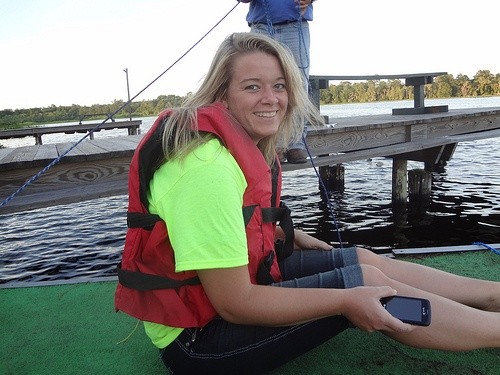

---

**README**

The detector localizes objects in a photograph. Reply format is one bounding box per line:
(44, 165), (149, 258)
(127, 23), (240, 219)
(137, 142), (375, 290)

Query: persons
(110, 34), (500, 375)
(239, 0), (315, 100)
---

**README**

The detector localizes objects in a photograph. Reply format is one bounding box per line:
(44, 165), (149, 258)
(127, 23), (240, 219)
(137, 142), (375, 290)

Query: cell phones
(380, 295), (432, 327)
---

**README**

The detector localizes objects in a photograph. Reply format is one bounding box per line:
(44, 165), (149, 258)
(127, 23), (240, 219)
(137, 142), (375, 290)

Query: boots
(275, 148), (286, 163)
(288, 148), (308, 162)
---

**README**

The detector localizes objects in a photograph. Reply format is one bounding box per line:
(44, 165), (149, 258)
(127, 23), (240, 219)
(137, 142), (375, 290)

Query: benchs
(78, 113), (115, 124)
(307, 72), (448, 125)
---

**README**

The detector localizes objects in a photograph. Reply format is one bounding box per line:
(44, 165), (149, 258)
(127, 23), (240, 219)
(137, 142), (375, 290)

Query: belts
(254, 19), (308, 26)
